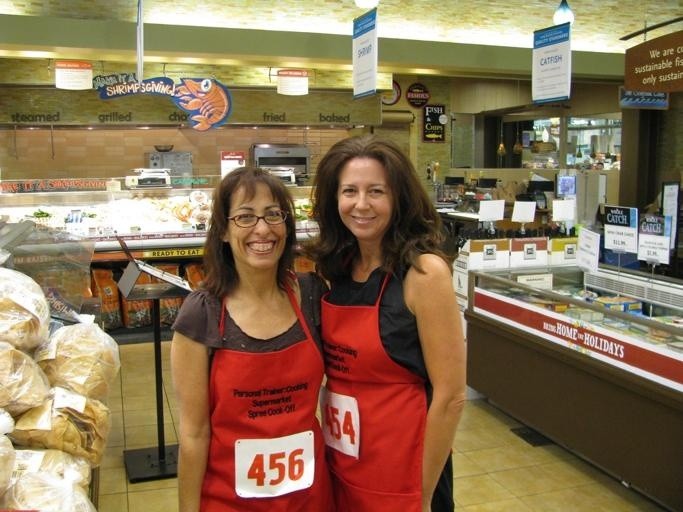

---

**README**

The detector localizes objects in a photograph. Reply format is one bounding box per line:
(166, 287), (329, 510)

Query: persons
(307, 132), (468, 511)
(167, 165), (334, 511)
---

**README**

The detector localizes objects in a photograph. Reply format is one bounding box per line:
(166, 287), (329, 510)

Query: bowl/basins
(153, 144), (173, 152)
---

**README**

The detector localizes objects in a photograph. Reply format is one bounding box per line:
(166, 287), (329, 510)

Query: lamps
(496, 114), (612, 159)
(553, 0), (575, 27)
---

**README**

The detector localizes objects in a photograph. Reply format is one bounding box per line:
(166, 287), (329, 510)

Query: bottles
(432, 160), (439, 183)
(455, 227), (577, 253)
(425, 164), (431, 181)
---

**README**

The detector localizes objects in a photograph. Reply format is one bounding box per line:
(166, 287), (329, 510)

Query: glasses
(227, 209), (290, 228)
